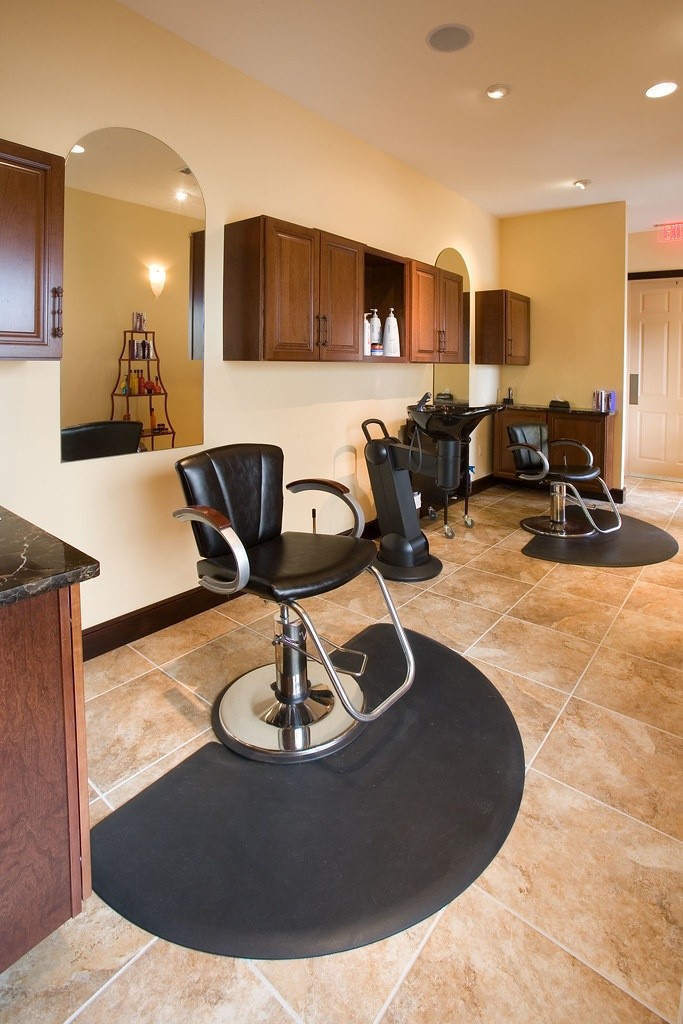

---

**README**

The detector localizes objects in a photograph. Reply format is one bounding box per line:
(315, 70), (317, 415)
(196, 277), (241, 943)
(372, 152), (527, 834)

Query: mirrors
(434, 248), (471, 408)
(61, 128), (206, 462)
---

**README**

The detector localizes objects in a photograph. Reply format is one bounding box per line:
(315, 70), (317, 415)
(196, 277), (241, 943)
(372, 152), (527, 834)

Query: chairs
(506, 420), (622, 538)
(173, 443), (415, 766)
(61, 421), (143, 463)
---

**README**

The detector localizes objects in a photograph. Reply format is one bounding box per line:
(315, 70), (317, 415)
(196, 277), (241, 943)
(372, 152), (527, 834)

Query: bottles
(150, 408), (156, 432)
(129, 370), (154, 394)
(120, 375), (131, 395)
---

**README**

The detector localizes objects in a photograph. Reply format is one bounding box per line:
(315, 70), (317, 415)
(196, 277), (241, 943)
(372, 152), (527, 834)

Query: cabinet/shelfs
(475, 289), (530, 366)
(222, 215), (464, 363)
(0, 139), (65, 359)
(110, 328), (177, 452)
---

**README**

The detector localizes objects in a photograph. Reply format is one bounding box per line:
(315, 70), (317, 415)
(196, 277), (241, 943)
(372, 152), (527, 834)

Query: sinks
(407, 404), (507, 441)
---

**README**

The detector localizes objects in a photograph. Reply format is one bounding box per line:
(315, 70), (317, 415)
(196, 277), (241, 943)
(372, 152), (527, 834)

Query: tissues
(436, 388), (453, 401)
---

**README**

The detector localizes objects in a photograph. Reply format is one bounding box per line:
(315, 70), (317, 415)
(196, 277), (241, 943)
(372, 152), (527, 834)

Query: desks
(0, 510), (101, 975)
(494, 405), (616, 494)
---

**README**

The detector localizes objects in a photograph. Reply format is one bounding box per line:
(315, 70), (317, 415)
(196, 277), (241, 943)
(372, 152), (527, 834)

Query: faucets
(415, 392), (432, 412)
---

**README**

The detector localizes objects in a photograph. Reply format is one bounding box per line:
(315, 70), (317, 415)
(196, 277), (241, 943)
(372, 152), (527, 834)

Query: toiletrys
(363, 308), (401, 357)
